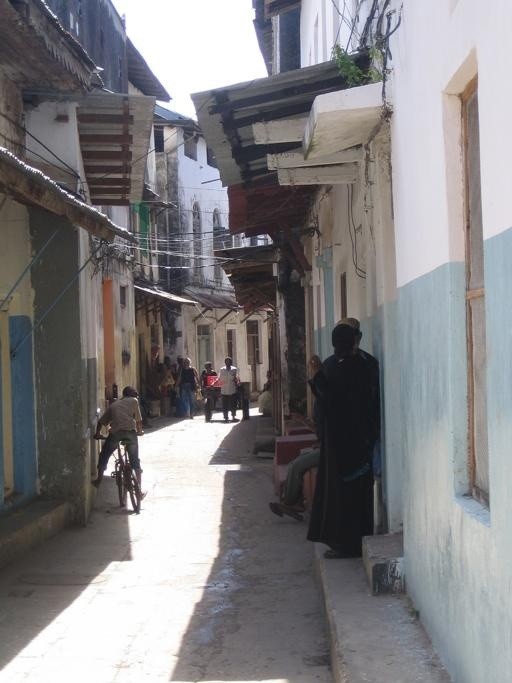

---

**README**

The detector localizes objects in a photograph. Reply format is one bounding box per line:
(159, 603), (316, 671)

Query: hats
(332, 318), (361, 331)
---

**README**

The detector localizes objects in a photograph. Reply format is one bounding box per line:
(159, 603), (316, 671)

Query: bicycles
(93, 431), (144, 514)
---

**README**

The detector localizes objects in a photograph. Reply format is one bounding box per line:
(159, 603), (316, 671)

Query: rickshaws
(202, 382), (250, 421)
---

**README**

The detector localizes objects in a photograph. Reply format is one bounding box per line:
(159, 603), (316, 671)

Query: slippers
(269, 502), (304, 521)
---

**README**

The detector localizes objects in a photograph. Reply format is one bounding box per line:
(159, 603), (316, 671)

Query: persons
(268, 409), (322, 520)
(91, 383), (148, 500)
(305, 322), (380, 560)
(312, 315), (380, 536)
(144, 354), (273, 423)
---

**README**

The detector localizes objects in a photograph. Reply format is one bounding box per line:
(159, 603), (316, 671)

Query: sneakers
(323, 547), (342, 559)
(92, 480), (100, 487)
(141, 490), (147, 499)
